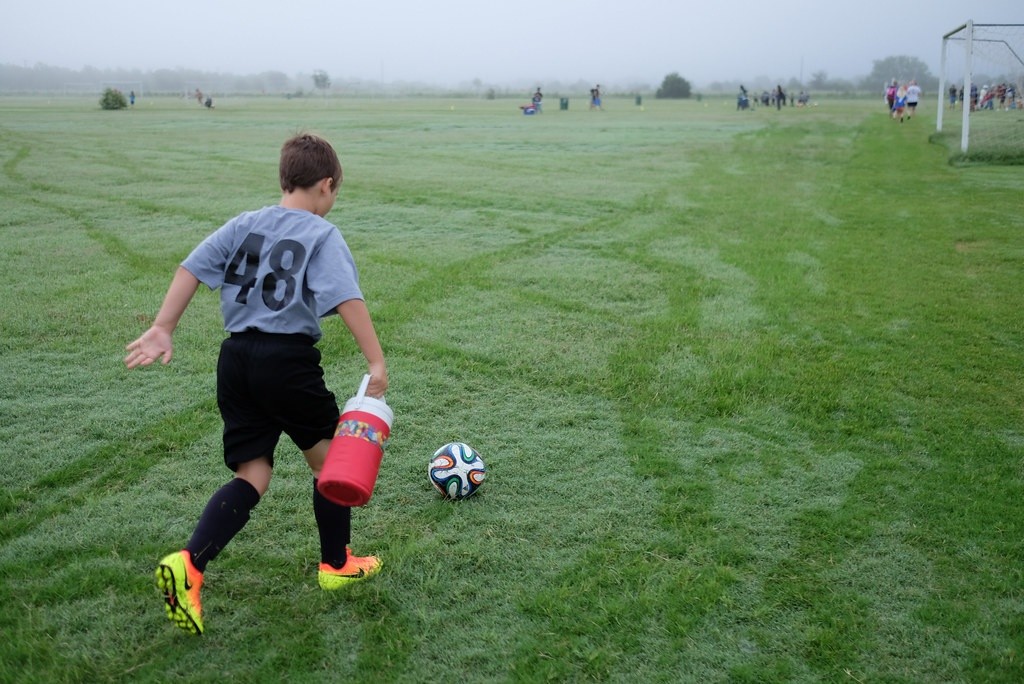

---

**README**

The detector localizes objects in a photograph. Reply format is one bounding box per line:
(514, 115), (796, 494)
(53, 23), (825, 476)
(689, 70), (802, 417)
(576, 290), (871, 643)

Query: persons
(126, 134), (388, 636)
(533, 87), (543, 114)
(195, 87), (204, 104)
(886, 78), (922, 122)
(205, 94), (212, 109)
(129, 91), (135, 111)
(949, 81), (1016, 111)
(590, 85), (605, 110)
(736, 85), (811, 111)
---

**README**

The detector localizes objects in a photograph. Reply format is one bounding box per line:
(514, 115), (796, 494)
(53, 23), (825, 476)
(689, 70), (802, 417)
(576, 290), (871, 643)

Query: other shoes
(1006, 108), (1008, 112)
(901, 118), (903, 123)
(908, 116), (910, 120)
(997, 109), (1000, 112)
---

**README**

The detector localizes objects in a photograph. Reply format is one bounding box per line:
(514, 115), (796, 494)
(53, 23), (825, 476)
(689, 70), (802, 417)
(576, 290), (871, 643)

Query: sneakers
(318, 546), (384, 590)
(154, 549), (206, 636)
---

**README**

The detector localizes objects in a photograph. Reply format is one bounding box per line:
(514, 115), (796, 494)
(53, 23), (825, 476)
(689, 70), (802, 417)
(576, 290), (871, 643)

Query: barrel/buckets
(318, 373), (394, 506)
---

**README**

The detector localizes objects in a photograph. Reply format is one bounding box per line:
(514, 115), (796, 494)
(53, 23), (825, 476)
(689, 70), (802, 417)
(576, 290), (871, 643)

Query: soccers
(426, 441), (487, 501)
